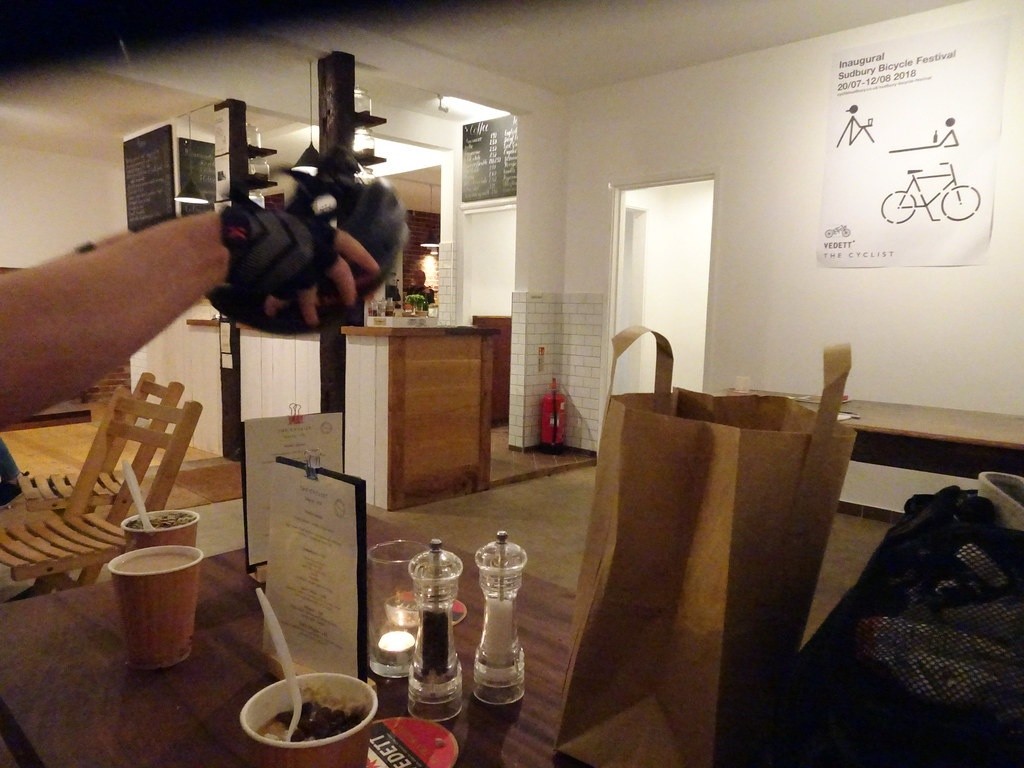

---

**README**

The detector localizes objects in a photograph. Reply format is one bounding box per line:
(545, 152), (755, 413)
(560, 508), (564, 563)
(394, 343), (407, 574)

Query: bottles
(354, 85), (371, 115)
(250, 155), (269, 181)
(368, 296), (394, 317)
(356, 165), (375, 185)
(246, 122), (261, 147)
(249, 188), (265, 210)
(473, 531), (527, 706)
(408, 539), (463, 722)
(354, 125), (374, 155)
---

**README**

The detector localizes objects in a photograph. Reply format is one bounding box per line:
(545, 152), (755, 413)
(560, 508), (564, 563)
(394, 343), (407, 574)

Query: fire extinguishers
(540, 376), (568, 454)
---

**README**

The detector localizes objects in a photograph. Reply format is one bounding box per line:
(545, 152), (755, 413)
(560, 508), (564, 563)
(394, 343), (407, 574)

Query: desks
(707, 384), (1023, 486)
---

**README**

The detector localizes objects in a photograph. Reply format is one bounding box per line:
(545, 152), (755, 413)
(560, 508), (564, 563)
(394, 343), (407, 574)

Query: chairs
(0, 383), (206, 589)
(0, 372), (188, 521)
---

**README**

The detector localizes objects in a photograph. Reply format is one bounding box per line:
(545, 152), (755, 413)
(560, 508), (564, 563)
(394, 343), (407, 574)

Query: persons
(406, 270), (435, 305)
(0, 196), (382, 443)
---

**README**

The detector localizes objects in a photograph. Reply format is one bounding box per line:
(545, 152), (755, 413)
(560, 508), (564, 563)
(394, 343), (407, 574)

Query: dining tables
(0, 512), (581, 767)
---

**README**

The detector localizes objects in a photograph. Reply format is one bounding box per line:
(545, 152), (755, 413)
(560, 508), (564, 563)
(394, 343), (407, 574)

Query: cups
(240, 672), (378, 768)
(108, 545), (204, 669)
(367, 539), (429, 678)
(122, 510), (200, 547)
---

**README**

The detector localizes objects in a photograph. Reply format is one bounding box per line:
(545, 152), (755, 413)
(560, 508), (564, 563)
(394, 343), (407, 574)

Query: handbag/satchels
(553, 315), (859, 768)
(795, 469), (1023, 768)
